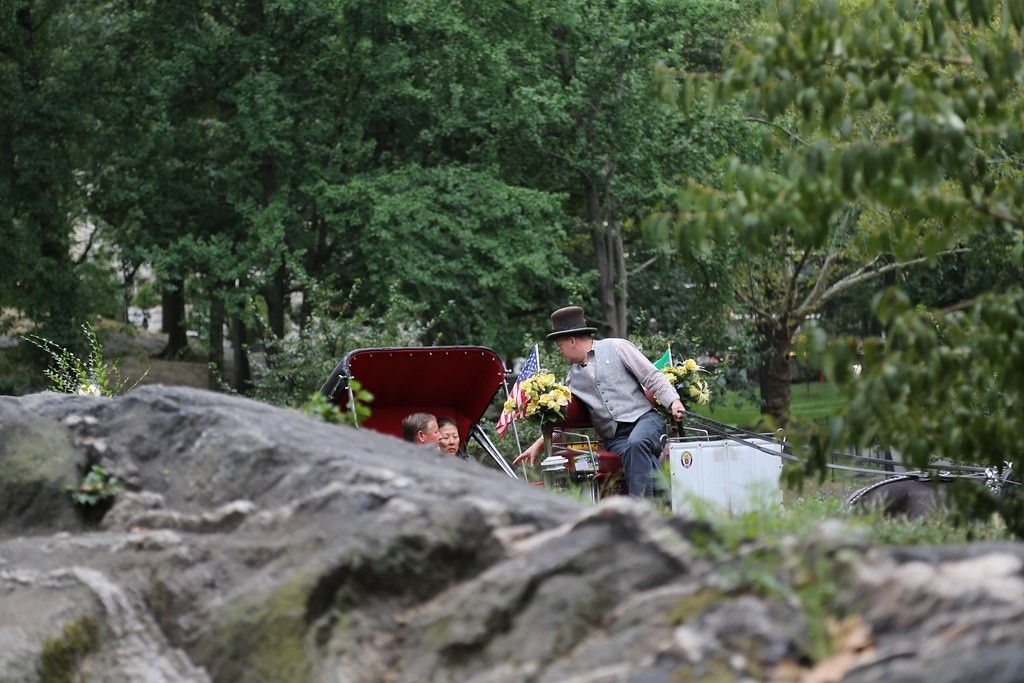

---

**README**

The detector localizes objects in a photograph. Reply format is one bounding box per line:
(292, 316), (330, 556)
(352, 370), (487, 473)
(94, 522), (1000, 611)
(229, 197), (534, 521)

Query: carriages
(312, 345), (1015, 527)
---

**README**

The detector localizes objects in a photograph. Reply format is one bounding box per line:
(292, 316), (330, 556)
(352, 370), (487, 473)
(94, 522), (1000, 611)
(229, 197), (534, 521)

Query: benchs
(364, 407), (472, 454)
(542, 391), (669, 479)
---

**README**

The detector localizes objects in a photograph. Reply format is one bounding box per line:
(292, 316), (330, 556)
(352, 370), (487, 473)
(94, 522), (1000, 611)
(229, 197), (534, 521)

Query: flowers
(506, 376), (573, 430)
(653, 358), (712, 409)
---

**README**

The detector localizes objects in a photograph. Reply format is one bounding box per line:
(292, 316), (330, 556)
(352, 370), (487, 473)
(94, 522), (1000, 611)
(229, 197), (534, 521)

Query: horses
(838, 467), (1024, 540)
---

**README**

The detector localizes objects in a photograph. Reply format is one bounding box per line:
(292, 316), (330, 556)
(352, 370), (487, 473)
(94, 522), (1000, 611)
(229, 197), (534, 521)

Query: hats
(545, 307), (597, 341)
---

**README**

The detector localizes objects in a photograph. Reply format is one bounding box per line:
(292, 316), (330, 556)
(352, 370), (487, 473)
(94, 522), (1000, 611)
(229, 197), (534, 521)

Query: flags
(639, 349), (675, 407)
(496, 343), (539, 437)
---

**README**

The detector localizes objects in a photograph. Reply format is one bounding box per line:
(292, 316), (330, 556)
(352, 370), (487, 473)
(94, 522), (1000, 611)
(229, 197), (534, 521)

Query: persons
(511, 306), (685, 504)
(402, 412), (479, 464)
(696, 349), (723, 365)
